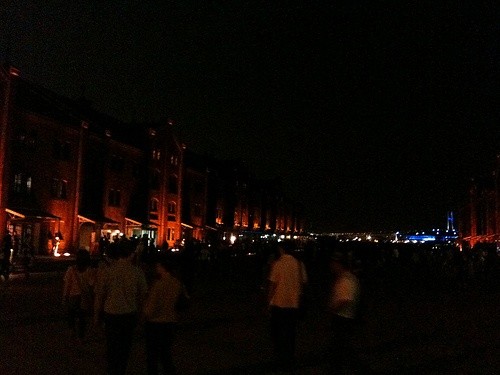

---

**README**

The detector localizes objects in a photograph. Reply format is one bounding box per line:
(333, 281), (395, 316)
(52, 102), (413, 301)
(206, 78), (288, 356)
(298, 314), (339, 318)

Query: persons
(323, 252), (364, 359)
(1, 219), (499, 321)
(267, 238), (309, 359)
(64, 248), (95, 347)
(97, 239), (147, 374)
(141, 249), (182, 374)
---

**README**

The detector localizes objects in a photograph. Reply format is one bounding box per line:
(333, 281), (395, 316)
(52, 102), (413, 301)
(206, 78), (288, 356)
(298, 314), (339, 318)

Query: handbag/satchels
(299, 284), (316, 318)
(176, 294), (191, 316)
(81, 289), (95, 310)
